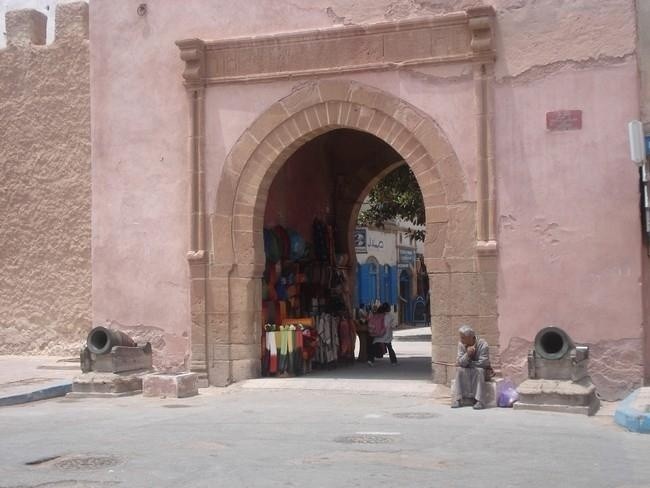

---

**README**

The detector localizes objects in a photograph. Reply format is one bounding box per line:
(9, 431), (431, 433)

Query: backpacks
(367, 311), (389, 337)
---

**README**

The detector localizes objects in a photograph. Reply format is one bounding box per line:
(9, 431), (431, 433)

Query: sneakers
(367, 360), (376, 368)
(391, 361), (401, 367)
(472, 401), (487, 410)
(451, 400), (465, 409)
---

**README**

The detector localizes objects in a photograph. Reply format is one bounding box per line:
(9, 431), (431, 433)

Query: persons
(359, 300), (399, 367)
(448, 325), (496, 409)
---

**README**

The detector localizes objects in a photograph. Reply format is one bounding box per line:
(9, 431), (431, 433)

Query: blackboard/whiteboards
(413, 297), (426, 323)
